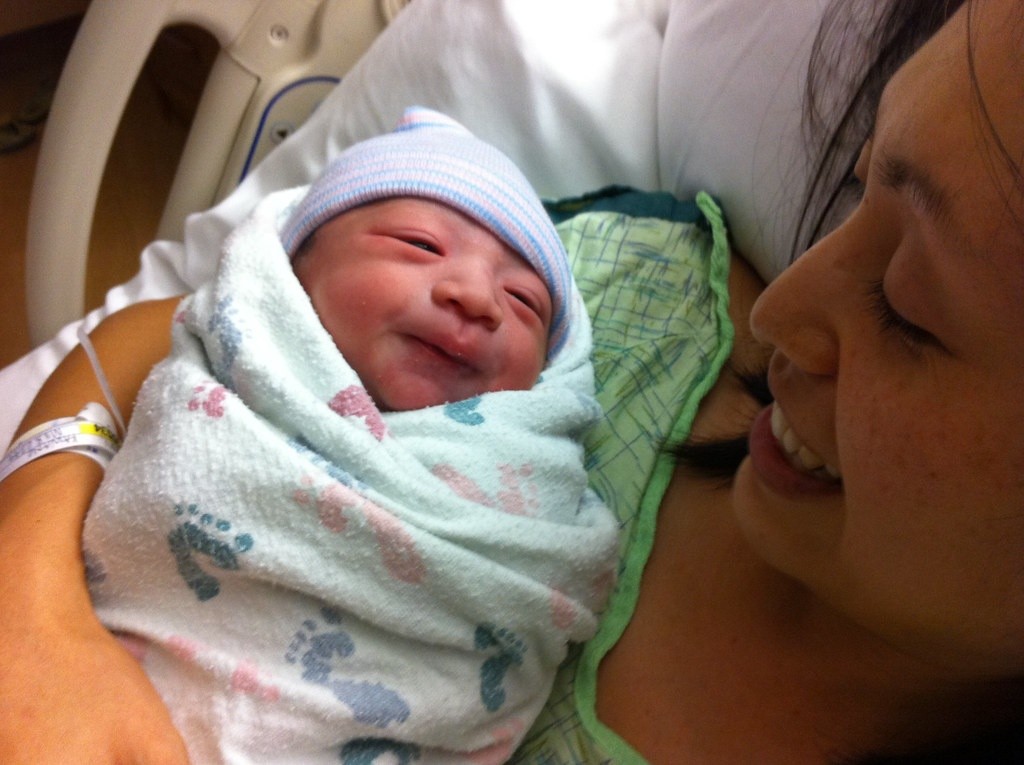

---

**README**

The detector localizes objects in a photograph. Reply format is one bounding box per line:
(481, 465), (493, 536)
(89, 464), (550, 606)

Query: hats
(281, 104), (570, 372)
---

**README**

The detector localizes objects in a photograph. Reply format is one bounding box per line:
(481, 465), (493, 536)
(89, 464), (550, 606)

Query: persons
(0, 0), (1024, 765)
(82, 103), (631, 765)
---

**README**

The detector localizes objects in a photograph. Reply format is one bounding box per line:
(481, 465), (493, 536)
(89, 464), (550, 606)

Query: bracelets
(0, 401), (123, 483)
